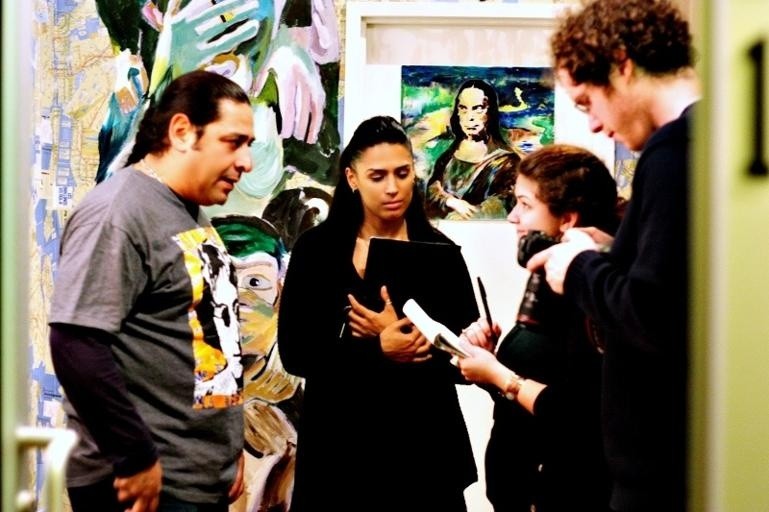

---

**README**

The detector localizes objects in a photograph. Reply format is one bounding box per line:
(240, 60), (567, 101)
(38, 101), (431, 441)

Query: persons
(523, 0), (707, 511)
(447, 146), (628, 511)
(277, 116), (480, 512)
(46, 72), (257, 512)
(423, 78), (522, 221)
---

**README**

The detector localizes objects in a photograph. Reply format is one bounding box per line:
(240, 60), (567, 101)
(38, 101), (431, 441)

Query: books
(402, 299), (469, 359)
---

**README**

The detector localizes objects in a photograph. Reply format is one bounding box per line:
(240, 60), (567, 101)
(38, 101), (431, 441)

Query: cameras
(514, 228), (562, 336)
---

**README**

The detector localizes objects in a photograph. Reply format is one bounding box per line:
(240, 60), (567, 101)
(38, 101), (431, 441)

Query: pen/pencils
(478, 277), (498, 345)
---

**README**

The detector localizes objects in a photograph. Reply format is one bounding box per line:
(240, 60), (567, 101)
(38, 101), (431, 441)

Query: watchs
(504, 376), (525, 400)
